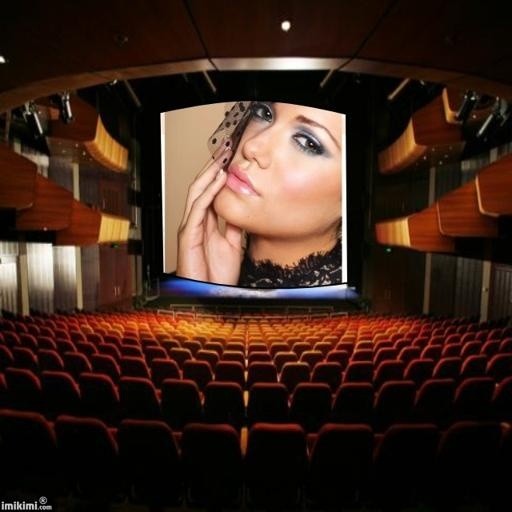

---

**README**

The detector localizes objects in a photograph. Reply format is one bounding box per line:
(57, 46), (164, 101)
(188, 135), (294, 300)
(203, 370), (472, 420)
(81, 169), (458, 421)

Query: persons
(171, 101), (342, 290)
